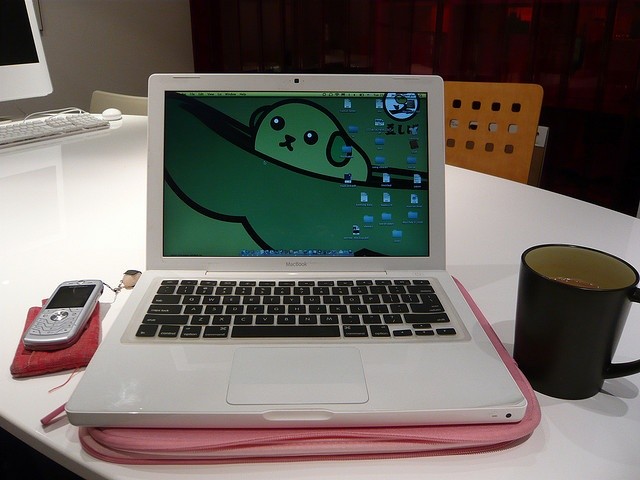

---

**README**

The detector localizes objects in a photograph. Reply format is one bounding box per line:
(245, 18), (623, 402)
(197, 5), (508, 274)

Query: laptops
(64, 73), (528, 429)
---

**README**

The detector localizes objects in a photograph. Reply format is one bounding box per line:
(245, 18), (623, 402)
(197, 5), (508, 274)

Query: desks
(0, 114), (640, 480)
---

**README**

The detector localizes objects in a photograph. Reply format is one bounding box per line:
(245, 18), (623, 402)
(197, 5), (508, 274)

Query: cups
(514, 243), (639, 400)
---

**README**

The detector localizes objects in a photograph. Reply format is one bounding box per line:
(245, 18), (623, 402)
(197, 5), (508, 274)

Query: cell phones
(22, 280), (104, 351)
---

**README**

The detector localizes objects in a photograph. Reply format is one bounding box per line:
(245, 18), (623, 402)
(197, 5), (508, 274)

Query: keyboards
(0, 112), (110, 150)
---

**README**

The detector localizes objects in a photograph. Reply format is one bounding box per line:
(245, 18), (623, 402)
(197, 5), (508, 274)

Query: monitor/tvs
(0, 1), (53, 125)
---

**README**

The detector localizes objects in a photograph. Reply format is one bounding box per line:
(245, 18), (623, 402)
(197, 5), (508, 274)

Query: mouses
(109, 119), (123, 129)
(102, 108), (122, 120)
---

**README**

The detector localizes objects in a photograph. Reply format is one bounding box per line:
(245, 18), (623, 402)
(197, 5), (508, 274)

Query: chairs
(88, 90), (149, 117)
(443, 79), (545, 185)
